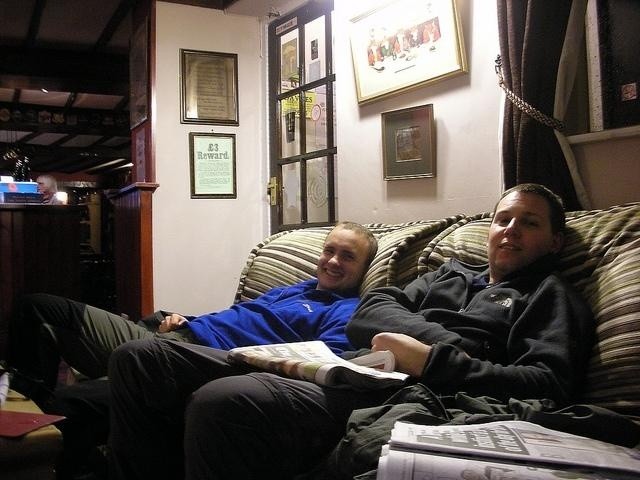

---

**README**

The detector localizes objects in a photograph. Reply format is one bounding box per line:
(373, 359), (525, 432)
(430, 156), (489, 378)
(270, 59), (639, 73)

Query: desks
(0, 385), (66, 479)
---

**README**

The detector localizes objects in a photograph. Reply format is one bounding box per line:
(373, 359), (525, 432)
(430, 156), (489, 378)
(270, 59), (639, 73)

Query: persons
(36, 173), (62, 205)
(14, 219), (379, 454)
(366, 19), (439, 63)
(107, 181), (595, 475)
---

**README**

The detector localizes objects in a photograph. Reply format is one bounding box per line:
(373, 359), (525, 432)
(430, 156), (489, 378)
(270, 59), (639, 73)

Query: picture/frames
(127, 12), (154, 132)
(346, 0), (470, 108)
(176, 47), (240, 128)
(187, 130), (237, 201)
(381, 103), (437, 181)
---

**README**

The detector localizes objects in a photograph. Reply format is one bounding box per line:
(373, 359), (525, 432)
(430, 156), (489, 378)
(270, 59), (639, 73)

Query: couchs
(417, 203), (640, 420)
(65, 213), (467, 419)
